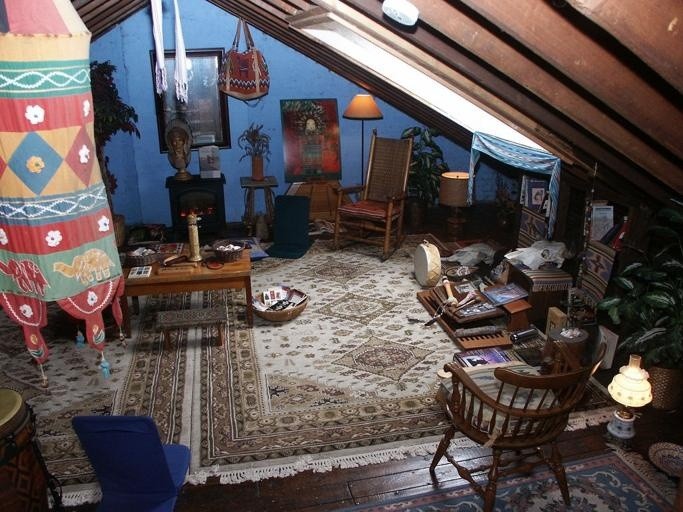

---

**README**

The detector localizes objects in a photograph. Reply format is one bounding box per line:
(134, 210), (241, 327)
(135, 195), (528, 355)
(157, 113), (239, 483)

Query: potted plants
(401, 126), (448, 226)
(238, 122), (272, 181)
(89, 60), (141, 247)
(596, 199), (683, 411)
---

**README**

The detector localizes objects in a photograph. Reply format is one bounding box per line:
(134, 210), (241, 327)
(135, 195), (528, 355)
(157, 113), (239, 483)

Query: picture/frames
(520, 174), (548, 210)
(149, 47), (232, 154)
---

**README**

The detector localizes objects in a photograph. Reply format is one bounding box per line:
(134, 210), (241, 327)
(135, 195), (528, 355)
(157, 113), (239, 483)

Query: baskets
(254, 291), (308, 322)
(213, 240), (245, 262)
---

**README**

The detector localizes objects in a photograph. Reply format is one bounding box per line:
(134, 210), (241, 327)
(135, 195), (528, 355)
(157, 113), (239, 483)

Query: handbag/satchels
(219, 20), (269, 100)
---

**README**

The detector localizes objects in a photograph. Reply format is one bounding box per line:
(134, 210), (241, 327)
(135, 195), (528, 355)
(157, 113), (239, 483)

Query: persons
(168, 131), (191, 167)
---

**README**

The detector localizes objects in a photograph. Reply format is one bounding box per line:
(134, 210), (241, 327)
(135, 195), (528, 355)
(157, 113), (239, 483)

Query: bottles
(510, 327), (538, 343)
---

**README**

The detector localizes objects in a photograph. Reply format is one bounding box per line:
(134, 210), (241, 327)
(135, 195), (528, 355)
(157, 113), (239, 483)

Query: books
(250, 285), (307, 312)
(590, 205), (615, 241)
(454, 347), (507, 368)
(481, 282), (530, 308)
(456, 302), (496, 318)
(519, 175), (550, 215)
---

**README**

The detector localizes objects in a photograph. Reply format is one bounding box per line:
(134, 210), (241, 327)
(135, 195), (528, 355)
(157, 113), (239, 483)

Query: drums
(0, 388), (50, 511)
(414, 242), (441, 287)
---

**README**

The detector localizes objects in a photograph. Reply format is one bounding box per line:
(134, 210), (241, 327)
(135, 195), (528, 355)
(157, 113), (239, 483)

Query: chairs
(72, 415), (190, 512)
(430, 343), (608, 511)
(333, 128), (414, 262)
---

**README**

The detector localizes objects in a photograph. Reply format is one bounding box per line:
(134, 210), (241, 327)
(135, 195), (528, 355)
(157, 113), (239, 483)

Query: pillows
(240, 238), (269, 262)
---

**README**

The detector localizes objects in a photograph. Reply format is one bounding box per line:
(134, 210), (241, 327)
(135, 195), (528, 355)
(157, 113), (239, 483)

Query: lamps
(607, 354), (653, 439)
(341, 95), (383, 186)
(439, 172), (470, 234)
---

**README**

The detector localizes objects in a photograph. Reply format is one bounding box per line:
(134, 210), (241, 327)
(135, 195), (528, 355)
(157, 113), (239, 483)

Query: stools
(156, 308), (226, 352)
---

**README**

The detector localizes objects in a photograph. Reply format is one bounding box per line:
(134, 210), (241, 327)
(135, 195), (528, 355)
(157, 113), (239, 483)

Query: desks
(240, 176), (279, 224)
(122, 242), (255, 338)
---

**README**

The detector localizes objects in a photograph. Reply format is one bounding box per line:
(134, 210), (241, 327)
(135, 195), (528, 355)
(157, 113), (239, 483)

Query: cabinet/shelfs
(165, 173), (226, 239)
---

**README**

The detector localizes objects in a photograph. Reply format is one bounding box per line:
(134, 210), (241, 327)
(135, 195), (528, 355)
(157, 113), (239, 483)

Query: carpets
(191, 233), (644, 484)
(0, 242), (198, 507)
(326, 448), (683, 512)
